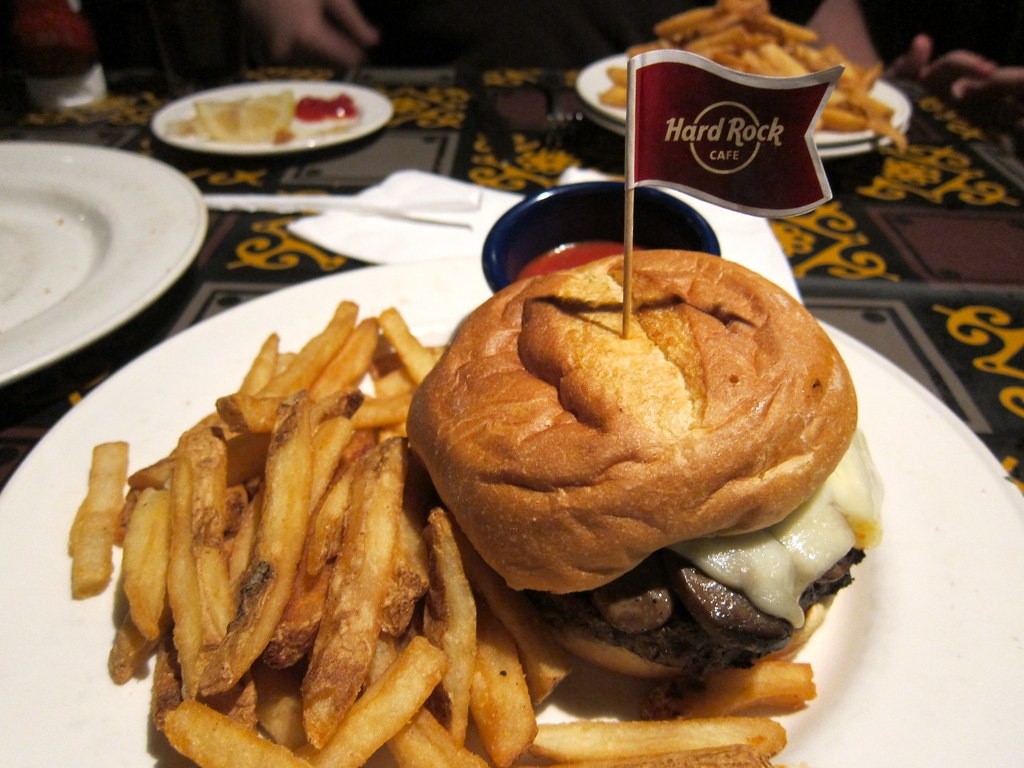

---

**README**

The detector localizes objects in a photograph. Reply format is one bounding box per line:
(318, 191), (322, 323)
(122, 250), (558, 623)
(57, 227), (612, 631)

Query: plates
(0, 254), (1024, 768)
(151, 81), (395, 155)
(0, 140), (209, 383)
(574, 51), (913, 161)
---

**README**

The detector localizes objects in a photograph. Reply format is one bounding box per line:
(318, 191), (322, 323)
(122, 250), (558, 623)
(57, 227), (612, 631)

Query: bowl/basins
(479, 180), (722, 299)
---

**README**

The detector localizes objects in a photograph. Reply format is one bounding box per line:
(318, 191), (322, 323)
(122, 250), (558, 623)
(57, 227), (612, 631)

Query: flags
(633, 49), (846, 220)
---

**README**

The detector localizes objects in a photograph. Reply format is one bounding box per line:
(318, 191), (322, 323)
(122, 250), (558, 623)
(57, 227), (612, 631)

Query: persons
(187, 0), (1024, 136)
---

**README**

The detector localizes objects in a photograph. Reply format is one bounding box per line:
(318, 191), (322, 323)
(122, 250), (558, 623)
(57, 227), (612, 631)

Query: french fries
(599, 1), (910, 149)
(68, 303), (814, 768)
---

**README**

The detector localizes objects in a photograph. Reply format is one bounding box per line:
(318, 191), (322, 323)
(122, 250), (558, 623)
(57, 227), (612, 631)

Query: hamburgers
(406, 248), (881, 680)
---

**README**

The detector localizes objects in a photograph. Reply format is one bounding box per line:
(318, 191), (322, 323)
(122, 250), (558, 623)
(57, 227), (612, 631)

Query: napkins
(286, 179), (524, 266)
(559, 164), (803, 309)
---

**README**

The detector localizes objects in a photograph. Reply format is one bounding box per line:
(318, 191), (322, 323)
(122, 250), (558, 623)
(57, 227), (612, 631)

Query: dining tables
(0, 68), (1023, 767)
